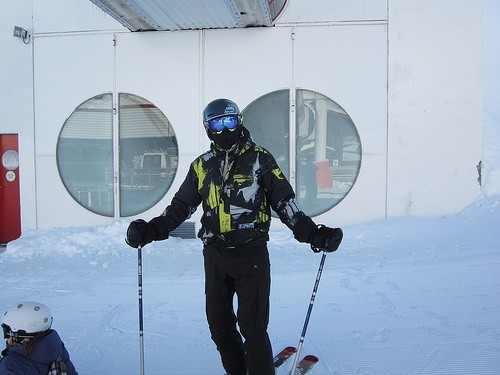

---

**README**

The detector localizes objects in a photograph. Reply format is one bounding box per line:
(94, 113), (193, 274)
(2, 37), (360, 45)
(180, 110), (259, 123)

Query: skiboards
(271, 345), (319, 375)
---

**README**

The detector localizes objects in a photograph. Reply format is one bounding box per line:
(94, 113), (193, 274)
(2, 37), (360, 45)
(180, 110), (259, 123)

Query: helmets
(202, 99), (242, 132)
(1, 302), (53, 343)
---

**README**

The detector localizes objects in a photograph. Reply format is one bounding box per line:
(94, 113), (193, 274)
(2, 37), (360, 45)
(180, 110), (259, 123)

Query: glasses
(205, 113), (241, 132)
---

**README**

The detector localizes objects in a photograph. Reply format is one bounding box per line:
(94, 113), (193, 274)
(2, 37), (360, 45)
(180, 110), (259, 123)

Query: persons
(0, 302), (80, 375)
(301, 154), (319, 208)
(125, 98), (343, 375)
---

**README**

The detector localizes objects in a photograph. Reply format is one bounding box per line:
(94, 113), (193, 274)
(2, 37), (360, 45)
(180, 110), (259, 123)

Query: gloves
(125, 215), (169, 249)
(293, 216), (343, 253)
